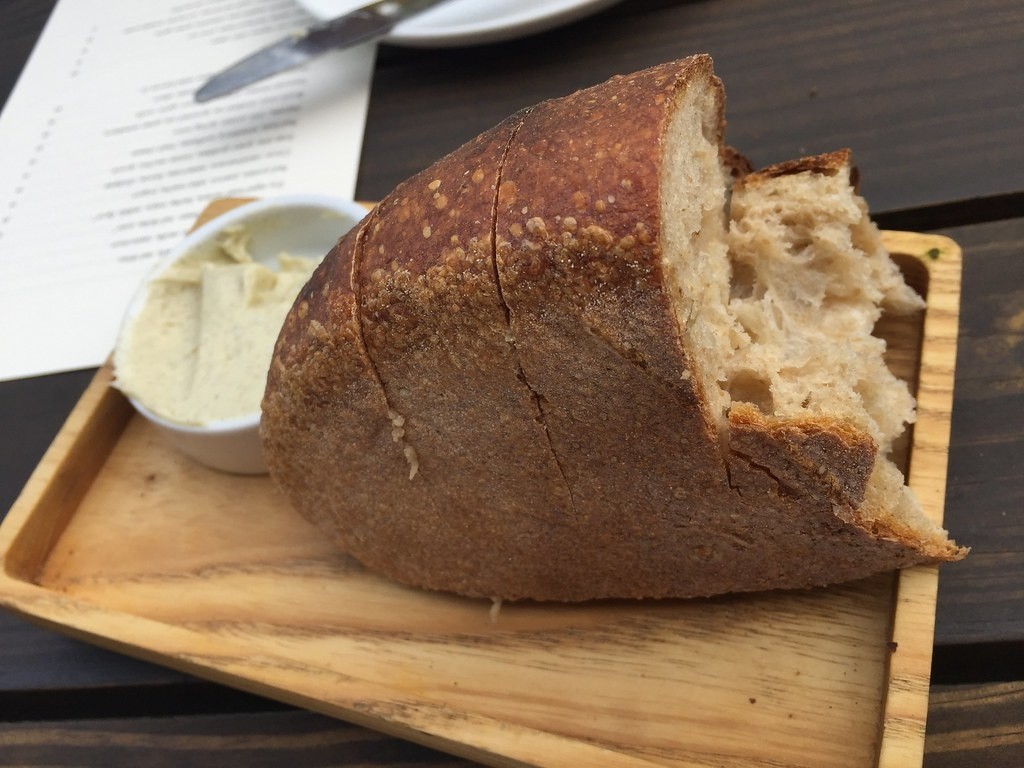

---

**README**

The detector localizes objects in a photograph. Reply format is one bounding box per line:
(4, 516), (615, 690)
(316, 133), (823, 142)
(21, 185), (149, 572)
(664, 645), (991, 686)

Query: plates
(297, 0), (614, 47)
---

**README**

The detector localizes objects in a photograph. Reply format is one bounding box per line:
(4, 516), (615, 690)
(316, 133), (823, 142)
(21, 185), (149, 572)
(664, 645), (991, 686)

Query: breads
(260, 54), (971, 603)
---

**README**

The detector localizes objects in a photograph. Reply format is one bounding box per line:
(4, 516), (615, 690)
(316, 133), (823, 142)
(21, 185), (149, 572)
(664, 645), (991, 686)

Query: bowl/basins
(115, 195), (370, 474)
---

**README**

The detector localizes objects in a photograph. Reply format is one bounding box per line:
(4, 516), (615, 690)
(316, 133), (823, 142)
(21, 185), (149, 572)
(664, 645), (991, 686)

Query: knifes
(195, 0), (446, 102)
